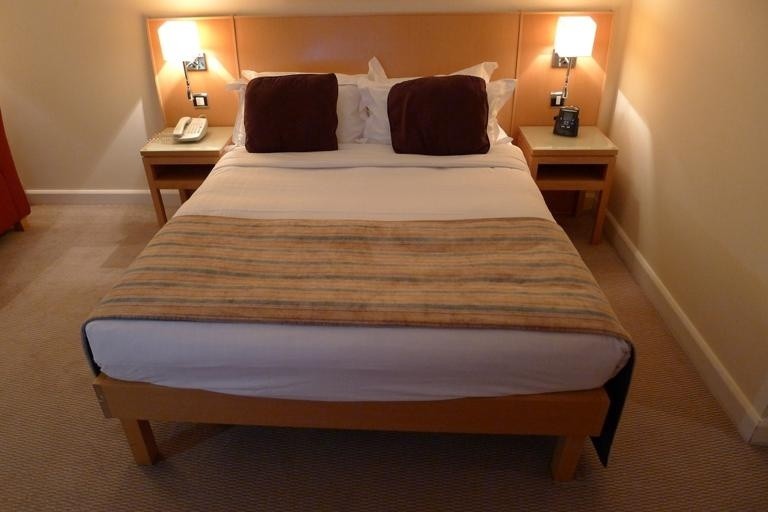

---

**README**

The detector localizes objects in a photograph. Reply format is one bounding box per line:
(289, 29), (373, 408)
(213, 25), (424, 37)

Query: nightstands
(520, 126), (619, 245)
(140, 127), (235, 228)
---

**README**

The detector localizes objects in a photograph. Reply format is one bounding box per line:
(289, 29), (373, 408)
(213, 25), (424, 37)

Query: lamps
(554, 16), (597, 99)
(156, 21), (200, 102)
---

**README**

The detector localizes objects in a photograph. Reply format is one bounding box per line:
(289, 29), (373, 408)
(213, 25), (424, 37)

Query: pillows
(228, 56), (517, 156)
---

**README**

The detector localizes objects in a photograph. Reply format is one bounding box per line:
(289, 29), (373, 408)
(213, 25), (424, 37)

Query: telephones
(173, 116), (208, 142)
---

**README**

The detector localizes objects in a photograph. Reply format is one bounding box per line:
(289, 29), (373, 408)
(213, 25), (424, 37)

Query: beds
(82, 9), (633, 486)
